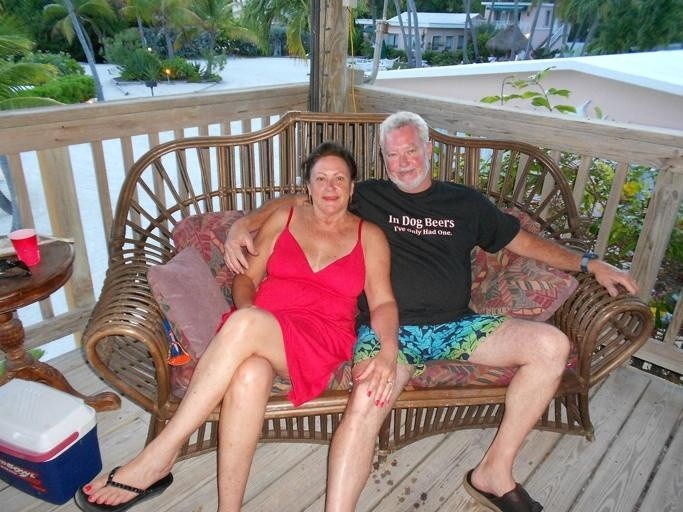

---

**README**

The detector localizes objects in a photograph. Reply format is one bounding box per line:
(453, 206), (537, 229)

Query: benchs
(79, 111), (656, 468)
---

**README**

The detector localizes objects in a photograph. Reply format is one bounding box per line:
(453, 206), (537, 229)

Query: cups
(8, 227), (40, 266)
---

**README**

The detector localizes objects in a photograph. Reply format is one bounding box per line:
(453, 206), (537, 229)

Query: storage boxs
(0, 374), (102, 505)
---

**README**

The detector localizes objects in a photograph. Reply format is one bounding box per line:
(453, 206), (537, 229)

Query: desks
(0, 232), (122, 414)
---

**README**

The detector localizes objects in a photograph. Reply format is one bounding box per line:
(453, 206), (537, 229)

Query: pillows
(470, 244), (579, 326)
(142, 244), (228, 366)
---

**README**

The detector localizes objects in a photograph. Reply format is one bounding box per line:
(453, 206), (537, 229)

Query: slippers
(463, 468), (544, 512)
(74, 465), (173, 512)
